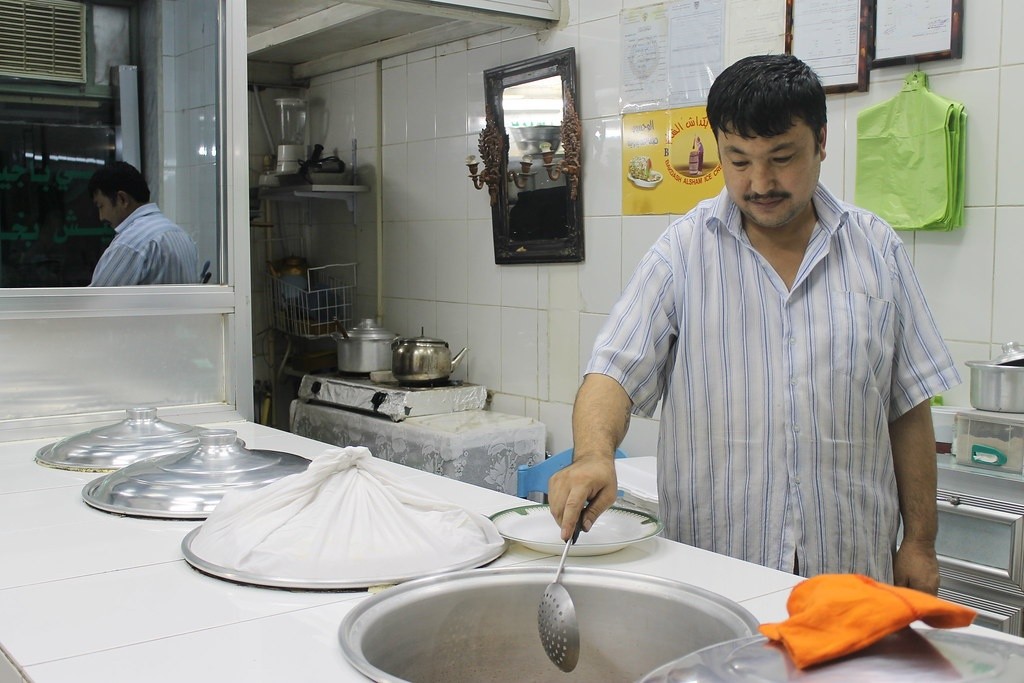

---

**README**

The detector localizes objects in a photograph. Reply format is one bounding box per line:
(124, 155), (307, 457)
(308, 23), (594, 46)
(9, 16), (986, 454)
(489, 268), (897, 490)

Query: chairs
(516, 443), (635, 517)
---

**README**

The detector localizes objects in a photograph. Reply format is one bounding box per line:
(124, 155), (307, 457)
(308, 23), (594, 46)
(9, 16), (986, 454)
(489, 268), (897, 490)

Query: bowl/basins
(965, 361), (1023, 415)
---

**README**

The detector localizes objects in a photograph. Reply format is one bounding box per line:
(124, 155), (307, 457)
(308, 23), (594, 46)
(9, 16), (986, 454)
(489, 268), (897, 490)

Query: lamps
(464, 154), (494, 192)
(508, 155), (532, 189)
(538, 142), (573, 181)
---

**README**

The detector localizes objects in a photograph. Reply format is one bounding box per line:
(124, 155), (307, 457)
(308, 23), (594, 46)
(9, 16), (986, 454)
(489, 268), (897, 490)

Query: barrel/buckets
(931, 406), (969, 454)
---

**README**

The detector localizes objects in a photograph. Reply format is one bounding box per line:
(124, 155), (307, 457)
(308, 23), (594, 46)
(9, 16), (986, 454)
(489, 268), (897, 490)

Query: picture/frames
(783, 0), (875, 99)
(869, 2), (964, 68)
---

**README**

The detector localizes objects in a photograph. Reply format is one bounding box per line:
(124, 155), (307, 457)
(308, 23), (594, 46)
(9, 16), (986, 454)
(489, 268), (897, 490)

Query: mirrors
(480, 46), (586, 268)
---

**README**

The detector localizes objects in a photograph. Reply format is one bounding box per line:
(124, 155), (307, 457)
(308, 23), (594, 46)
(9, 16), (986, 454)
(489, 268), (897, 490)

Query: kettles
(390, 327), (468, 383)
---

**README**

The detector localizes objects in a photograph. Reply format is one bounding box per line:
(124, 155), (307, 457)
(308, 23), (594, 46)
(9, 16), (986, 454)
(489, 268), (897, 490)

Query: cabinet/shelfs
(287, 396), (547, 509)
(895, 457), (1024, 640)
(254, 176), (384, 394)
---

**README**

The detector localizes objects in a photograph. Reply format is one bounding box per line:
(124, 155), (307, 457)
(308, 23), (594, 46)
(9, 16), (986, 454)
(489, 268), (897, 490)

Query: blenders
(274, 97), (308, 174)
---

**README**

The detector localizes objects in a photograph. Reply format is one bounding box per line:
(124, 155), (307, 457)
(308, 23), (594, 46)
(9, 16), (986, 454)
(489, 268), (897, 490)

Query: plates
(488, 504), (664, 558)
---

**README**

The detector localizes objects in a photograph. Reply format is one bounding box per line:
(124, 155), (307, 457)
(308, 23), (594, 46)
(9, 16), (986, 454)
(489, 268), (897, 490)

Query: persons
(544, 53), (963, 602)
(86, 162), (202, 286)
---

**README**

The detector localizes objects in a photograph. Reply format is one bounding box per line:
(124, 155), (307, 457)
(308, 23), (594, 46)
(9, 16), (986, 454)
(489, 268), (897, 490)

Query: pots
(330, 318), (395, 372)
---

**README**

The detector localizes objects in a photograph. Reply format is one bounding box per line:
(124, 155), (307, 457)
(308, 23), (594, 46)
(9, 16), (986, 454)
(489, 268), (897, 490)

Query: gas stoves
(297, 372), (488, 422)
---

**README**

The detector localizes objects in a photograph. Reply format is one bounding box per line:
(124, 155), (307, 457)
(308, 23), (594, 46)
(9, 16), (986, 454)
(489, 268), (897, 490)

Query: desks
(1, 419), (1024, 683)
